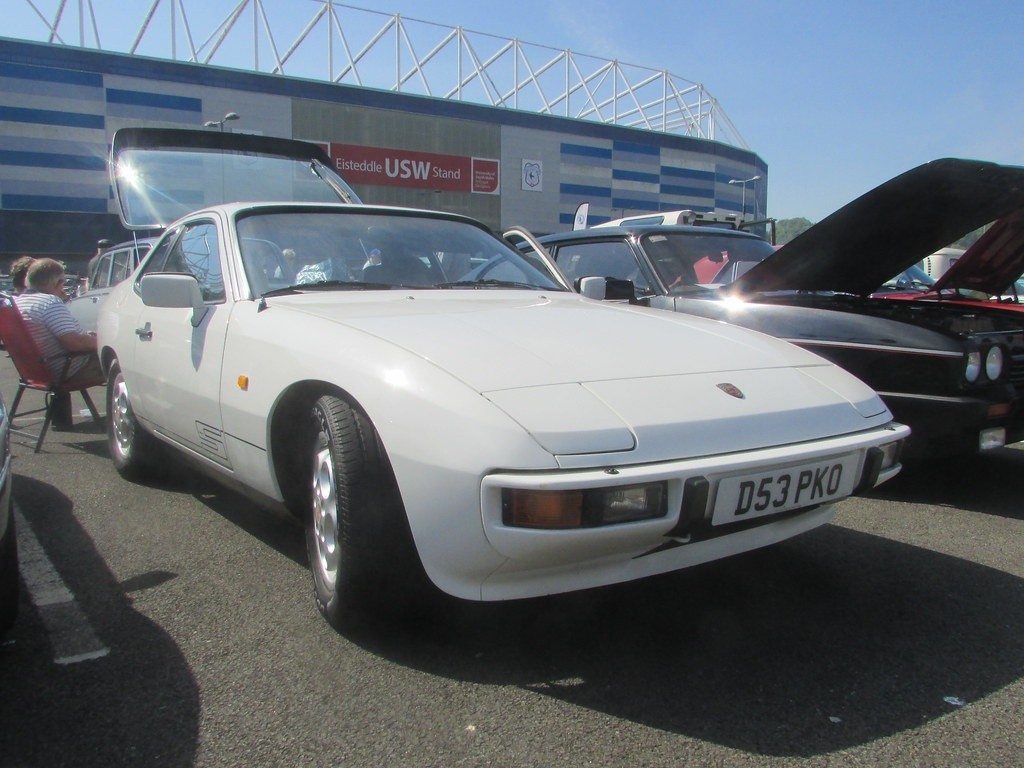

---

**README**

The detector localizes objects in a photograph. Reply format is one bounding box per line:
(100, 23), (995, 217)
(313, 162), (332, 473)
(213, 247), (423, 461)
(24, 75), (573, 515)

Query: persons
(86, 239), (115, 292)
(437, 251), (473, 281)
(5, 256), (104, 385)
(274, 249), (297, 280)
(363, 248), (382, 270)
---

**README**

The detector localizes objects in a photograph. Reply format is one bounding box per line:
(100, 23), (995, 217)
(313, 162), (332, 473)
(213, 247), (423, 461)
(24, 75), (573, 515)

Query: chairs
(0, 293), (106, 453)
(364, 226), (433, 284)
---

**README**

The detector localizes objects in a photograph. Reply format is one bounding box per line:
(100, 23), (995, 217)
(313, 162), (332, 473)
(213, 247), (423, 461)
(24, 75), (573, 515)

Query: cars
(65, 235), (295, 333)
(570, 209), (1024, 345)
(457, 157), (1024, 464)
(96, 127), (913, 633)
(0, 394), (20, 641)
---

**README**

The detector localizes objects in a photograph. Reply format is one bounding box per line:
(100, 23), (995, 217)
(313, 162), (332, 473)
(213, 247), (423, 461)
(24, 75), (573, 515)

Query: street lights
(729, 176), (761, 219)
(204, 112), (241, 204)
